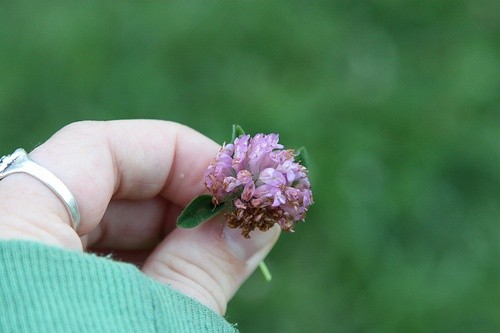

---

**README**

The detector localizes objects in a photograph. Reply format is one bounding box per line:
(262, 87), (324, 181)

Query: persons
(0, 119), (281, 333)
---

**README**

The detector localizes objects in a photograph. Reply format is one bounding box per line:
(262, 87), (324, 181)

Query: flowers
(175, 123), (316, 282)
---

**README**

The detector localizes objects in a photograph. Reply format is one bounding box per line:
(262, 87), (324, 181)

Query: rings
(0, 147), (81, 234)
